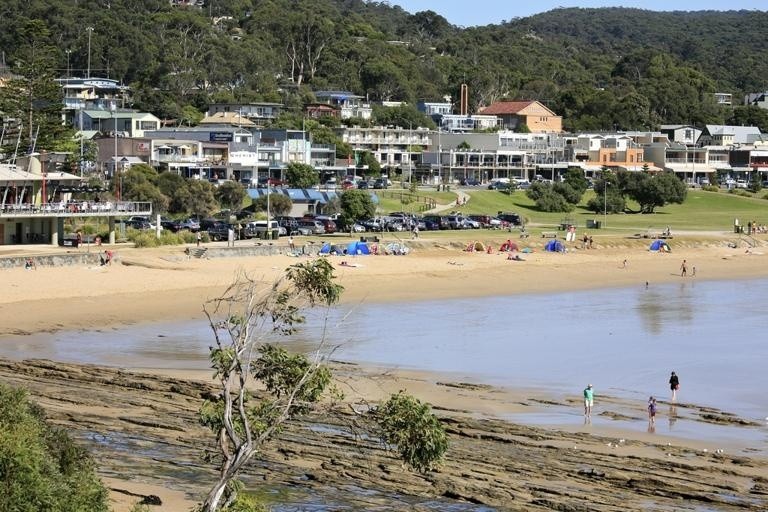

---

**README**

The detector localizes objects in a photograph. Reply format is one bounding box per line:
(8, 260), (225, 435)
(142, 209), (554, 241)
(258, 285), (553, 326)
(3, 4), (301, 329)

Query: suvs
(272, 214), (337, 234)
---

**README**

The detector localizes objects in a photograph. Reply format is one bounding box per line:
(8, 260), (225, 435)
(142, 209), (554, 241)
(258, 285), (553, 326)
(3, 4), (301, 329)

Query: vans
(426, 215), (449, 229)
(243, 220), (279, 238)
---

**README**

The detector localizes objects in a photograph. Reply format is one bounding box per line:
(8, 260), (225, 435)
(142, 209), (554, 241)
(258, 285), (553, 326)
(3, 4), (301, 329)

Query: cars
(128, 215), (151, 230)
(488, 175), (566, 190)
(412, 217), (439, 230)
(59, 233), (80, 247)
(363, 217), (411, 232)
(461, 178), (481, 185)
(443, 215), (479, 229)
(209, 177), (391, 189)
(762, 180), (768, 187)
(7, 197), (135, 211)
(160, 217), (201, 234)
(203, 219), (246, 242)
(702, 177), (748, 187)
(114, 220), (131, 227)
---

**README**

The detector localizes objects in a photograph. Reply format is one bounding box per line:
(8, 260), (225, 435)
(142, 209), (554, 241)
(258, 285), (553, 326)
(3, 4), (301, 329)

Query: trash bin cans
(586, 219), (595, 229)
(597, 222), (601, 228)
(260, 231), (267, 239)
(273, 231), (278, 239)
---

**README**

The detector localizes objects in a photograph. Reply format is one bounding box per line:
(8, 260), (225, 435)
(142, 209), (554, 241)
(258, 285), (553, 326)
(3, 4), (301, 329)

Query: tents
(317, 239), (409, 255)
(467, 239), (566, 253)
(650, 239), (671, 251)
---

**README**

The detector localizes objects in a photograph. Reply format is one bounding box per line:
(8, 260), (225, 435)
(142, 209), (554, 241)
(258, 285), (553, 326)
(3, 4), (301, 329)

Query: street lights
(604, 181), (611, 230)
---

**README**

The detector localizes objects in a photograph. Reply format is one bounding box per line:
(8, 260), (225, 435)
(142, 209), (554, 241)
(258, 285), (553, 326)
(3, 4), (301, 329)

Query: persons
(664, 225), (671, 236)
(455, 195), (466, 206)
(583, 235), (594, 248)
(680, 259), (696, 277)
(488, 246), (492, 254)
(48, 198), (98, 213)
(196, 230), (203, 246)
(669, 371), (679, 401)
(105, 249), (112, 265)
(733, 216), (768, 235)
(95, 235), (101, 248)
(412, 222), (420, 239)
(582, 384), (593, 419)
(659, 246), (665, 254)
(566, 226), (576, 241)
(648, 396), (657, 424)
(287, 236), (295, 249)
(623, 260), (628, 270)
(25, 256), (37, 272)
